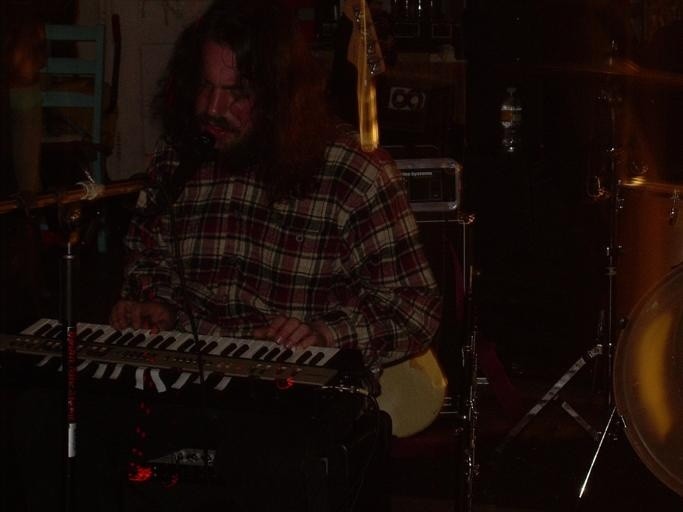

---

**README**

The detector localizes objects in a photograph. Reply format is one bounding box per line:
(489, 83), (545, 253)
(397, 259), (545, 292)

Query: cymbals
(548, 59), (679, 82)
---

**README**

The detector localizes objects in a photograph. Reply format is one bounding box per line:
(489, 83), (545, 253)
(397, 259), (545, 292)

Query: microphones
(141, 131), (217, 214)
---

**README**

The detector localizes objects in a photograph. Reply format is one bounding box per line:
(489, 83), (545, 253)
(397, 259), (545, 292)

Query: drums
(613, 263), (681, 497)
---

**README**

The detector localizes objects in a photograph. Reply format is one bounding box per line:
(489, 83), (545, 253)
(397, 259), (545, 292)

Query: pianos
(2, 318), (389, 512)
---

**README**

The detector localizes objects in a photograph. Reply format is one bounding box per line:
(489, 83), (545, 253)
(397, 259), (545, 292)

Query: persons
(107, 0), (445, 511)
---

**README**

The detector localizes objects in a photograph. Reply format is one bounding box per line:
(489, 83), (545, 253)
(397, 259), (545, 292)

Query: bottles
(498, 87), (526, 152)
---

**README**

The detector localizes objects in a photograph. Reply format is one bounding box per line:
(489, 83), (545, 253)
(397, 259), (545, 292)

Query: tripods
(508, 252), (624, 498)
